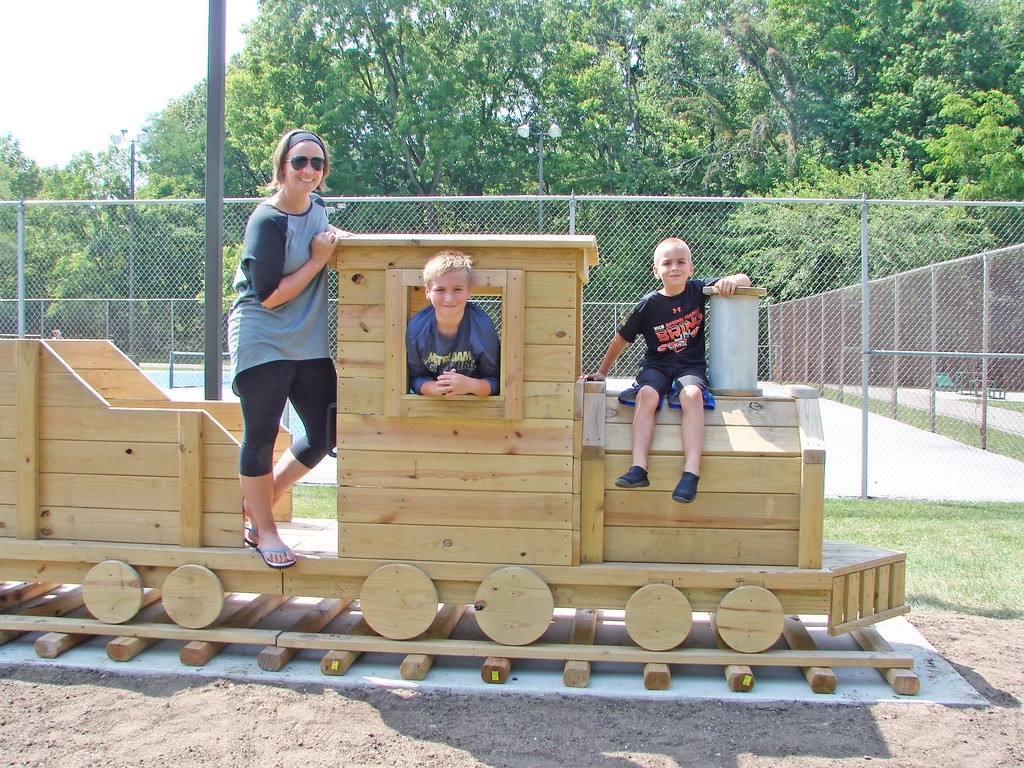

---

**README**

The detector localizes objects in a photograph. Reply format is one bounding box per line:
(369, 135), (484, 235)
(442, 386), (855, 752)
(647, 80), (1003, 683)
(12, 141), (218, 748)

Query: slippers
(243, 526), (259, 547)
(254, 548), (296, 567)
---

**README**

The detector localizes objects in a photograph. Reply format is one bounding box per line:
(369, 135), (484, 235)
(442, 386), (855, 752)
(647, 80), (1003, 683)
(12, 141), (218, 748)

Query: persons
(404, 250), (502, 400)
(580, 238), (751, 502)
(225, 130), (353, 568)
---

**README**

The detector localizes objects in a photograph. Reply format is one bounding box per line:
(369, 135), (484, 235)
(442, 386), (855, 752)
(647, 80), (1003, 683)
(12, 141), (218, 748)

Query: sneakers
(615, 465), (652, 488)
(672, 473), (701, 504)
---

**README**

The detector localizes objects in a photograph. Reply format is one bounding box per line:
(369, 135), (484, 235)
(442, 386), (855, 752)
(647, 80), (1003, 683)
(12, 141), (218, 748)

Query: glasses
(285, 156), (326, 171)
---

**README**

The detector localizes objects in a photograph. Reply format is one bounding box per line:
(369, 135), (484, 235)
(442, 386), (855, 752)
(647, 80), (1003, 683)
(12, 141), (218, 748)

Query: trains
(0, 231), (909, 655)
